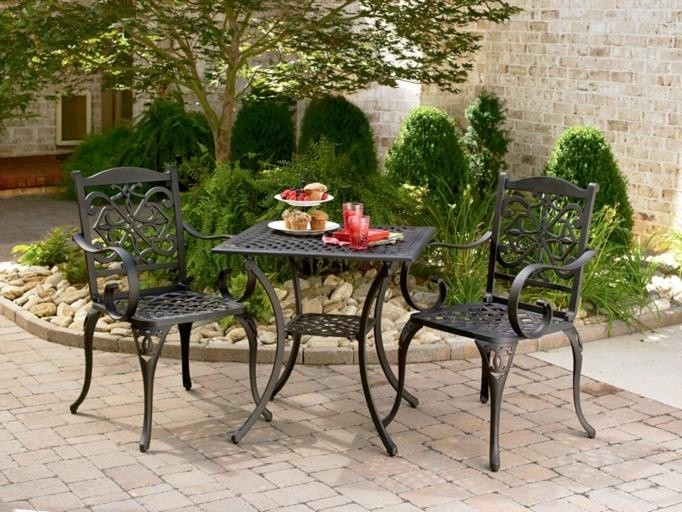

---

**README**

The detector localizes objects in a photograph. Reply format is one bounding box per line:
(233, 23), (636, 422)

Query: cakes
(310, 209), (329, 230)
(281, 208), (300, 229)
(304, 182), (327, 201)
(288, 211), (311, 230)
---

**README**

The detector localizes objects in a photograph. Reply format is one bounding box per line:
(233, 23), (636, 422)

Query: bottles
(300, 180), (310, 214)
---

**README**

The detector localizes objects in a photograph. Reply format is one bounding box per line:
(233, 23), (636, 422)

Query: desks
(212, 218), (440, 458)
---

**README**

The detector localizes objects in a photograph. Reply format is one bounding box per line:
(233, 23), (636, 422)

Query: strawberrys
(281, 188), (327, 201)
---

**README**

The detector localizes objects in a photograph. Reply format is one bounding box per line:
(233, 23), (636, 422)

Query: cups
(342, 201), (363, 232)
(347, 215), (369, 251)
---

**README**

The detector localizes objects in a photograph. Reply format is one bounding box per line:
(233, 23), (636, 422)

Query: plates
(267, 220), (341, 236)
(331, 228), (390, 243)
(274, 192), (334, 207)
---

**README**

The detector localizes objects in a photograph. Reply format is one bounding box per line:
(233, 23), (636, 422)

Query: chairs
(371, 170), (601, 474)
(68, 163), (273, 452)
